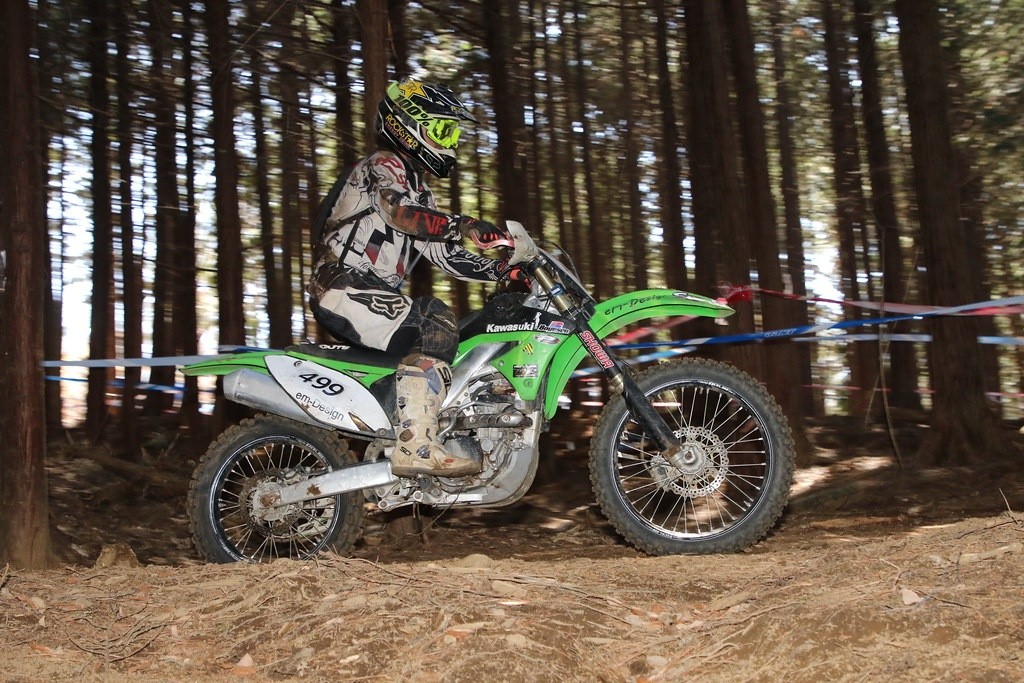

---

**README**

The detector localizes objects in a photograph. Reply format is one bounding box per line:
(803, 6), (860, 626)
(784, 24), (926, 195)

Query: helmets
(373, 75), (482, 179)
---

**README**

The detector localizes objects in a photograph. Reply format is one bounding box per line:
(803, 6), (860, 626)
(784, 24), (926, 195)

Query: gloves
(499, 261), (531, 288)
(455, 215), (512, 251)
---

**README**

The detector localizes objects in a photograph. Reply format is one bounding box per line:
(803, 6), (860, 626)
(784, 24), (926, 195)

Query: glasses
(387, 82), (460, 149)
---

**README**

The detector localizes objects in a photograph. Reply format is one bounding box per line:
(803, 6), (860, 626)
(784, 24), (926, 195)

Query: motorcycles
(178, 220), (795, 567)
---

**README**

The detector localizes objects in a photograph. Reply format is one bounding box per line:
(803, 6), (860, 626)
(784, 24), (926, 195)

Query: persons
(305, 80), (515, 479)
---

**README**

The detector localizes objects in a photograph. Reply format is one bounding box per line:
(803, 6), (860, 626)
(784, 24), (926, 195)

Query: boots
(392, 353), (481, 476)
